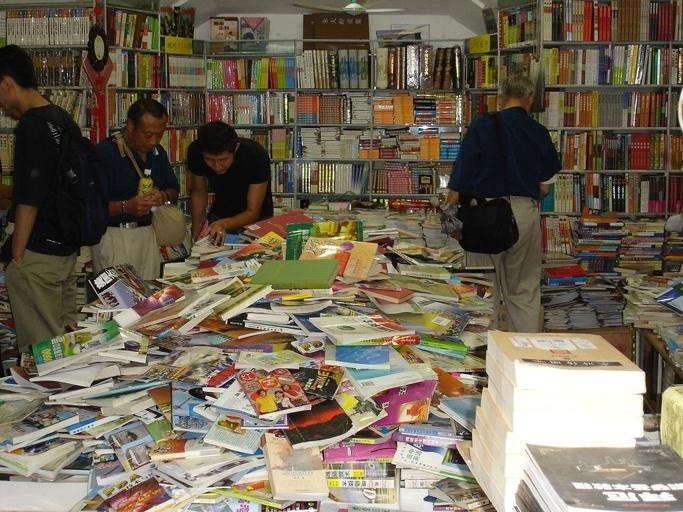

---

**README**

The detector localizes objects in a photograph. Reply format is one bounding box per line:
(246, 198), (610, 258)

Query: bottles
(138, 167), (153, 201)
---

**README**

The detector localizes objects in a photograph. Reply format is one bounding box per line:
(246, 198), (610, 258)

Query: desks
(633, 326), (683, 419)
(562, 323), (655, 414)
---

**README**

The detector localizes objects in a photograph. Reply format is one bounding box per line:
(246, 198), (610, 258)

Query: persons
(89, 97), (181, 285)
(126, 432), (153, 469)
(73, 328), (108, 345)
(255, 384), (304, 414)
(446, 75), (560, 336)
(227, 31), (238, 50)
(1, 44), (82, 366)
(185, 121), (276, 248)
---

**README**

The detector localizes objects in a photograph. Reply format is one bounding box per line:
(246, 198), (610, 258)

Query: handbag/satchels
(153, 205), (186, 247)
(454, 199), (519, 254)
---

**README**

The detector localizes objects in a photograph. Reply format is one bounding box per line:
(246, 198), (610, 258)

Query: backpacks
(62, 137), (110, 246)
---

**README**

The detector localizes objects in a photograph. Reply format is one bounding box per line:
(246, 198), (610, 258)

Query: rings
(218, 229), (222, 235)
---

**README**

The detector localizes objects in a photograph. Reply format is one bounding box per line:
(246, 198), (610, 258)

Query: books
(1, 1), (683, 511)
